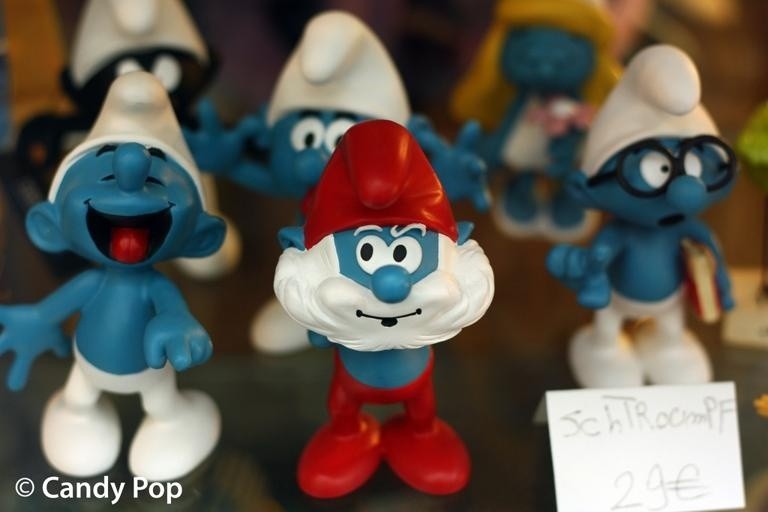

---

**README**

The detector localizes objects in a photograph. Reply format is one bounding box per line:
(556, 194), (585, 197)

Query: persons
(0, 1), (740, 500)
